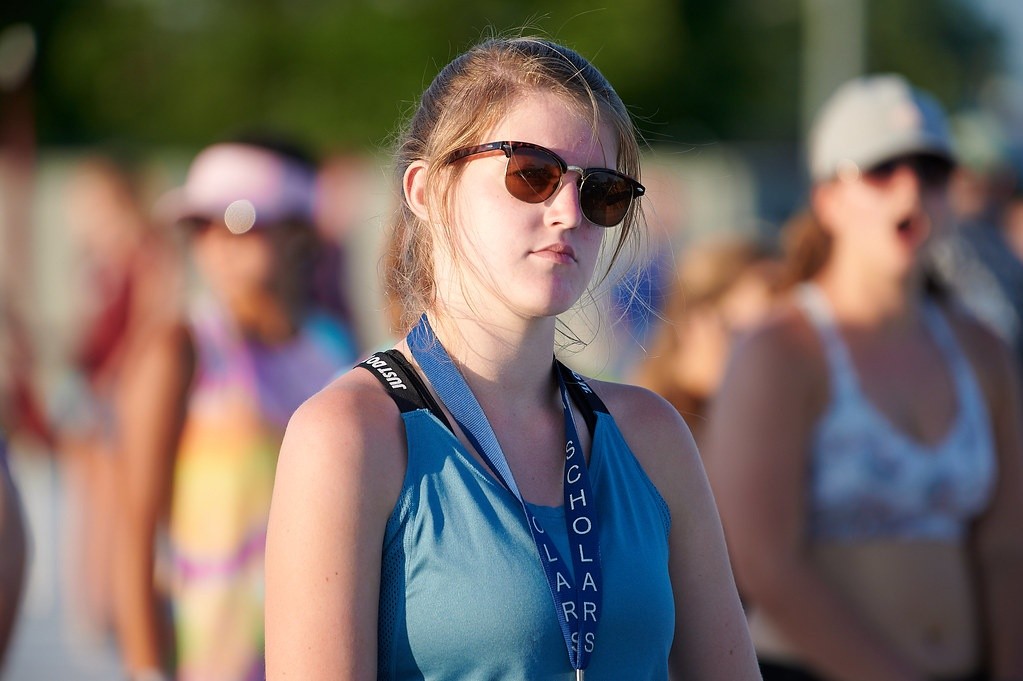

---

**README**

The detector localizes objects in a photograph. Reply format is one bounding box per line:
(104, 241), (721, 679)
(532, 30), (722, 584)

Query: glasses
(861, 154), (954, 186)
(445, 141), (645, 227)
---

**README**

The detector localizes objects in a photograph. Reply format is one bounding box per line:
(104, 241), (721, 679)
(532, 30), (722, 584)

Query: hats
(153, 142), (313, 226)
(807, 72), (955, 183)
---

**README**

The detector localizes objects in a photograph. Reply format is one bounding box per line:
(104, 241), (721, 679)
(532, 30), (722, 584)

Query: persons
(264, 35), (765, 681)
(638, 74), (1023, 681)
(0, 3), (362, 681)
